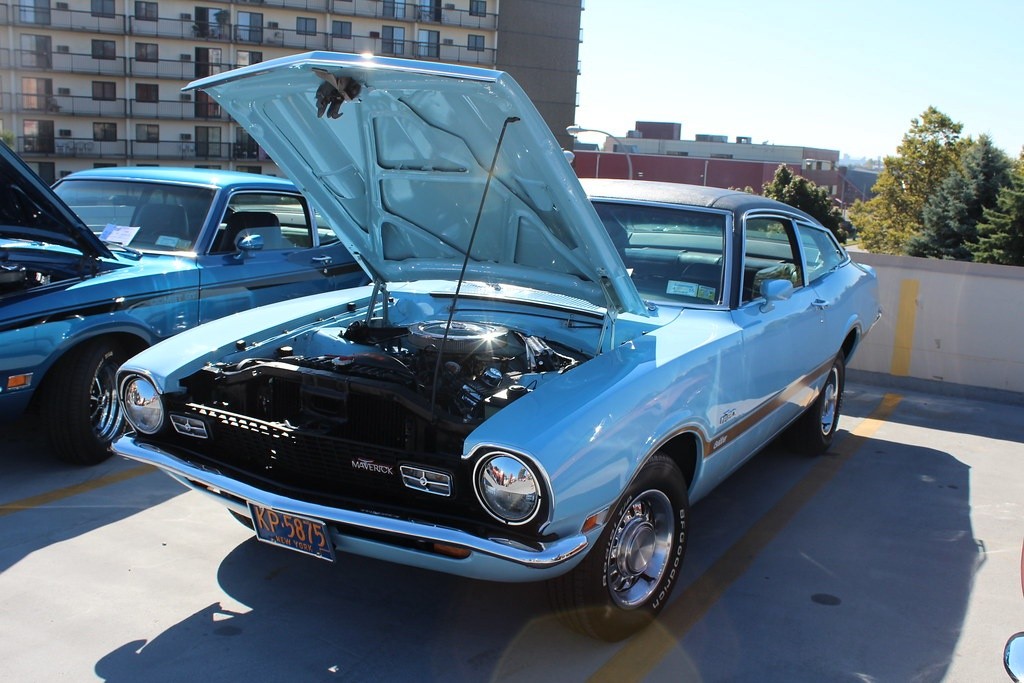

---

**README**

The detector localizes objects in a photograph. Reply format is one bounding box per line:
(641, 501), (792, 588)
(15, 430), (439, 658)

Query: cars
(0, 133), (372, 469)
(103, 49), (882, 643)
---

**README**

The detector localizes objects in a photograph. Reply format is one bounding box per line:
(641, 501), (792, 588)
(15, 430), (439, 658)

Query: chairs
(134, 204), (188, 244)
(221, 211), (281, 257)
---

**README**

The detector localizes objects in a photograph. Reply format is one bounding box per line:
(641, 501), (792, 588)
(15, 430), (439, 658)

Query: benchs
(623, 249), (798, 308)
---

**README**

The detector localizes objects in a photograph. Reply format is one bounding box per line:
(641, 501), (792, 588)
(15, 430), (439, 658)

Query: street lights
(804, 158), (846, 216)
(565, 123), (634, 180)
